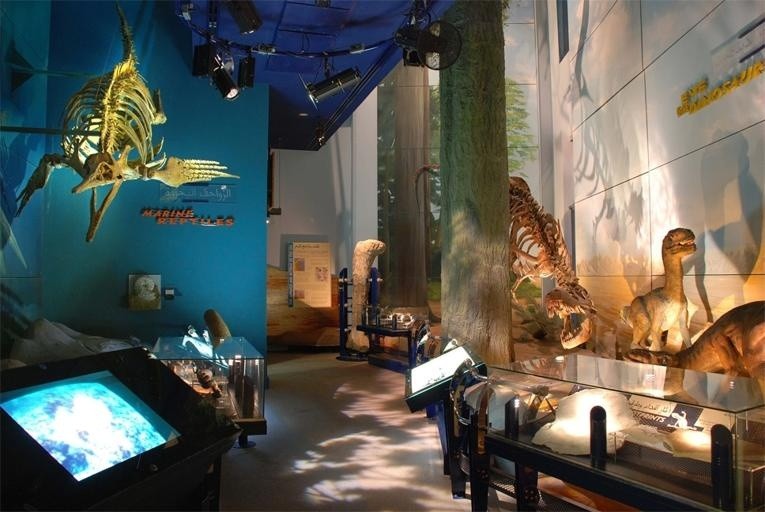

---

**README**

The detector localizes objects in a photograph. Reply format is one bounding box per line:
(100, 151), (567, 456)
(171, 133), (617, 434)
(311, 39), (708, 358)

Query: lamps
(172, 0), (370, 108)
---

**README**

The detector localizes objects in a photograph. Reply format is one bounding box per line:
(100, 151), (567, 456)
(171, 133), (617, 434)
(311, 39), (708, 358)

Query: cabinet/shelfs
(337, 268), (429, 375)
(148, 332), (270, 447)
(467, 343), (764, 510)
